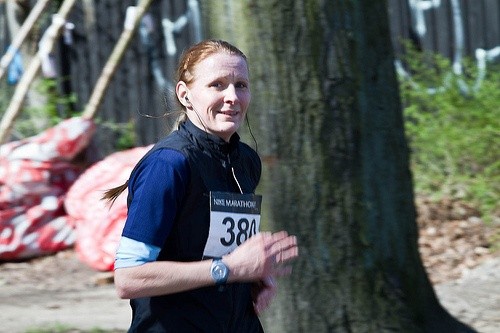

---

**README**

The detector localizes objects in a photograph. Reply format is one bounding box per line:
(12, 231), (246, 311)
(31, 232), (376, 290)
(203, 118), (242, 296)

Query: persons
(114, 36), (299, 333)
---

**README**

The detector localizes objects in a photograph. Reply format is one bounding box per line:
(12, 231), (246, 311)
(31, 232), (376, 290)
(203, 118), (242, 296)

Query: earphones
(185, 96), (191, 104)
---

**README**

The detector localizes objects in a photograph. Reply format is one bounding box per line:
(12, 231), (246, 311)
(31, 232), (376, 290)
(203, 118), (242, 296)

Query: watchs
(210, 256), (229, 291)
